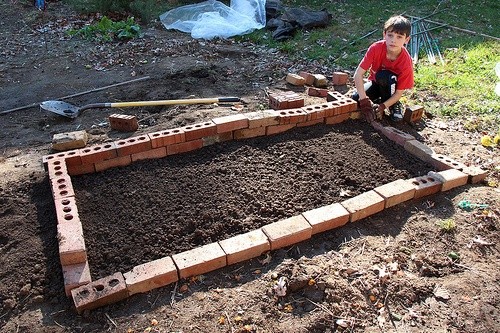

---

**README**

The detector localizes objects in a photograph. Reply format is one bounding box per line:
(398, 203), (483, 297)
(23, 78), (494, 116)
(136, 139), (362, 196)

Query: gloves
(359, 96), (386, 122)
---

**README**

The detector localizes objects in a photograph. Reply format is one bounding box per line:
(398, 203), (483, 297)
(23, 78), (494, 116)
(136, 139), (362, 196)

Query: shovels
(39, 95), (240, 119)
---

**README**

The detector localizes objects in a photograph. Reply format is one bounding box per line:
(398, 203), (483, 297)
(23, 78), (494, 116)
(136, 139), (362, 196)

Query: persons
(352, 16), (414, 124)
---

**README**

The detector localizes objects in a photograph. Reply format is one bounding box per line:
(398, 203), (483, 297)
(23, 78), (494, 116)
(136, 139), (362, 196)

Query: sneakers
(389, 102), (403, 118)
